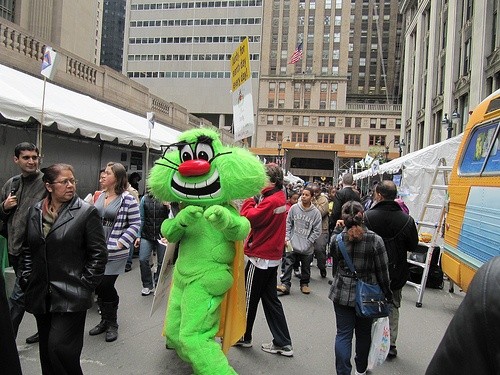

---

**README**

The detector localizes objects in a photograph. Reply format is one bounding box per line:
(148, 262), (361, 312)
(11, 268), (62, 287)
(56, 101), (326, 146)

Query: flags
(357, 149), (380, 174)
(289, 44), (303, 64)
(41, 47), (56, 79)
(147, 112), (155, 129)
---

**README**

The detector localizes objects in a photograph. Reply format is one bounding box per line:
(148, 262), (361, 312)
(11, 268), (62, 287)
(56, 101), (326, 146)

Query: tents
(352, 132), (464, 243)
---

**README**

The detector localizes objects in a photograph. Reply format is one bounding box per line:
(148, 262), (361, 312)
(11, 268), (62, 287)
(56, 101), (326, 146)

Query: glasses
(51, 178), (79, 186)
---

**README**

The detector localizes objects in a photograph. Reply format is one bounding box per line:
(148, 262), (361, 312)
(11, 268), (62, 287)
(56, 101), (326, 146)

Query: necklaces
(106, 191), (115, 199)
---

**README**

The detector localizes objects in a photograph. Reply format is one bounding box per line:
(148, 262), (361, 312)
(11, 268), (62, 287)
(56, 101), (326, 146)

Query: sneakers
(261, 339), (294, 356)
(142, 286), (155, 295)
(220, 336), (253, 347)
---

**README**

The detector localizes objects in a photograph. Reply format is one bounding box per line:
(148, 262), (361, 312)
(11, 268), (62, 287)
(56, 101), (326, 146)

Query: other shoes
(320, 268), (326, 278)
(26, 332), (39, 344)
(276, 284), (291, 295)
(97, 300), (101, 314)
(354, 366), (368, 375)
(294, 270), (301, 277)
(387, 348), (397, 357)
(300, 285), (311, 293)
(125, 264), (132, 272)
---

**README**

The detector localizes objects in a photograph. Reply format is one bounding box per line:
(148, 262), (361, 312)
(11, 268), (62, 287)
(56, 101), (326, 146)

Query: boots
(89, 297), (107, 336)
(103, 300), (120, 342)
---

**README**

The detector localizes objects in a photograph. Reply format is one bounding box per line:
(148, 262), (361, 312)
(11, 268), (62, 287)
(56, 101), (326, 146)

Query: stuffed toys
(146, 127), (272, 375)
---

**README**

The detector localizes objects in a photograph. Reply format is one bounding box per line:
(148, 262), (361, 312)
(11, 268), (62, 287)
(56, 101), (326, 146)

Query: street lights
(276, 143), (282, 165)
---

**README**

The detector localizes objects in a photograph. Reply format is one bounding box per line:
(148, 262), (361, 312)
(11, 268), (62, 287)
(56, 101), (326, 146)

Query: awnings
(0, 63), (183, 151)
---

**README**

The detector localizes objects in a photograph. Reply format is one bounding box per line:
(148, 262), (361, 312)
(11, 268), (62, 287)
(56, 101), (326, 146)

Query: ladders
(406, 157), (454, 308)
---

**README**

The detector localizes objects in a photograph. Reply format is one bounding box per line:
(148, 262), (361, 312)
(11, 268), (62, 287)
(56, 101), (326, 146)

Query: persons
(327, 201), (397, 375)
(221, 162), (294, 356)
(0, 142), (109, 375)
(365, 181), (409, 214)
(425, 256), (500, 375)
(83, 162), (142, 342)
(125, 173), (180, 296)
(363, 180), (420, 361)
(277, 170), (362, 294)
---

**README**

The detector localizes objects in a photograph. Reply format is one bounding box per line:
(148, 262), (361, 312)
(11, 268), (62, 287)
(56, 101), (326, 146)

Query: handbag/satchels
(367, 315), (391, 371)
(355, 277), (390, 319)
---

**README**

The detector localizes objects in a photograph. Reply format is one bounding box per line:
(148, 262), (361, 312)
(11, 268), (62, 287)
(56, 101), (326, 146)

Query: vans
(439, 86), (500, 294)
